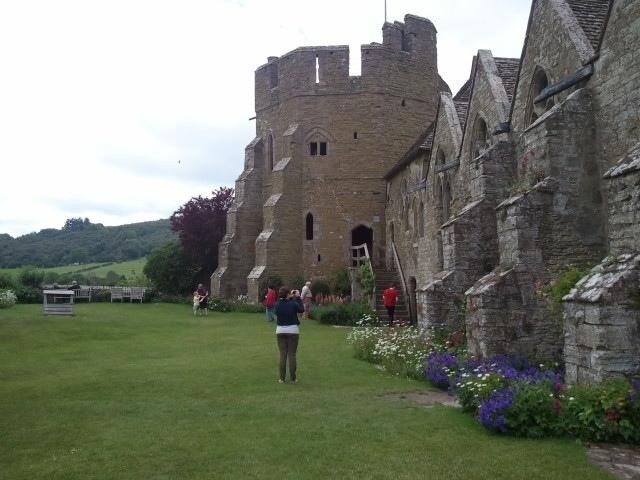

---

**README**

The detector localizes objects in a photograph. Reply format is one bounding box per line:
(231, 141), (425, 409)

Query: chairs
(111, 287), (144, 304)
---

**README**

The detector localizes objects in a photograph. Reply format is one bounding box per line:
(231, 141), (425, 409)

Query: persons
(382, 282), (400, 329)
(272, 286), (304, 382)
(49, 282), (65, 303)
(192, 291), (205, 316)
(197, 283), (209, 316)
(66, 280), (80, 303)
(263, 286), (276, 322)
(301, 281), (312, 319)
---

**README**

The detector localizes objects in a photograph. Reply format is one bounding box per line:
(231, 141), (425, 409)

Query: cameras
(293, 291), (296, 294)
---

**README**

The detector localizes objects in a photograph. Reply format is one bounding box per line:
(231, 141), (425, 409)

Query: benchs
(72, 288), (94, 303)
